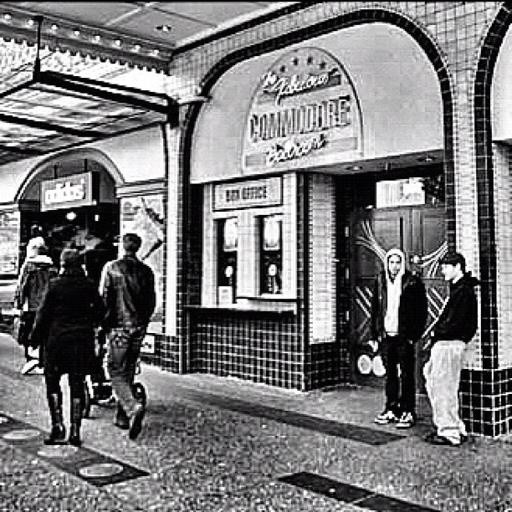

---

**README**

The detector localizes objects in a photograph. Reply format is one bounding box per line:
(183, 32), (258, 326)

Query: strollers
(77, 325), (147, 419)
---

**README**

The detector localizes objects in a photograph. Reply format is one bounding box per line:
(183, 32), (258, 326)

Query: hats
(438, 252), (465, 266)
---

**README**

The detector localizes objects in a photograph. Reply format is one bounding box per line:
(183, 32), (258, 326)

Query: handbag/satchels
(13, 317), (27, 344)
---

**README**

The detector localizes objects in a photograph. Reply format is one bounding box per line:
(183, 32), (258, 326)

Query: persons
(12, 235), (54, 375)
(414, 252), (478, 448)
(31, 247), (106, 449)
(369, 248), (428, 430)
(95, 233), (155, 440)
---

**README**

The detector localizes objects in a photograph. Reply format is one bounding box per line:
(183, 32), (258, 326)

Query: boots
(44, 393), (65, 444)
(68, 397), (85, 445)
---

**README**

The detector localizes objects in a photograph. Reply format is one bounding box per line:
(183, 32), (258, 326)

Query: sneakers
(395, 410), (417, 429)
(424, 431), (468, 446)
(129, 402), (145, 439)
(20, 359), (41, 375)
(112, 417), (128, 428)
(374, 406), (399, 425)
